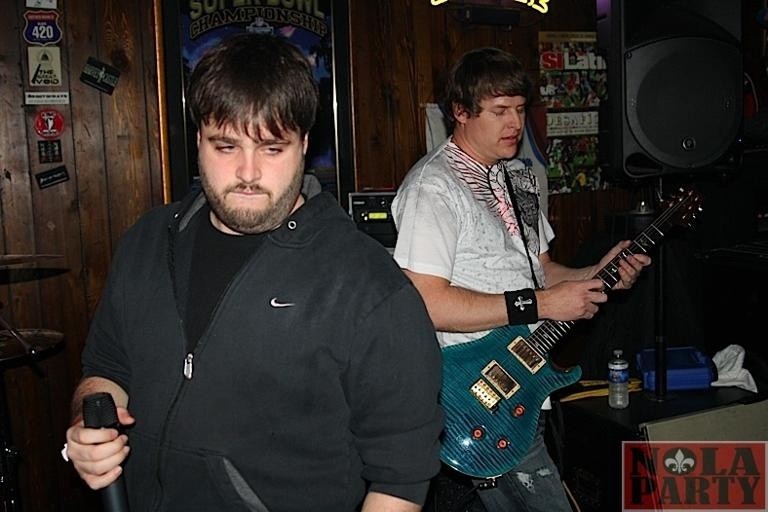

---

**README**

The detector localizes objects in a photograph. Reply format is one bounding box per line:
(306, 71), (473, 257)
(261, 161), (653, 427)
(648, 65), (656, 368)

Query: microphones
(81, 392), (130, 512)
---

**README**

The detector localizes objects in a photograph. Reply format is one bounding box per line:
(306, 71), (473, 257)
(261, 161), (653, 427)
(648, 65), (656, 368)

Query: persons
(539, 74), (599, 177)
(59, 32), (446, 512)
(388, 43), (653, 511)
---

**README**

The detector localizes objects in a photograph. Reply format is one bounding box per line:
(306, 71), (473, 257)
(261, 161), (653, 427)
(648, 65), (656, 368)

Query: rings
(60, 442), (69, 462)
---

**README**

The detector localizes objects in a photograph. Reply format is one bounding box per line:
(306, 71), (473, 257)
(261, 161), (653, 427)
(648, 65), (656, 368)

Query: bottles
(607, 350), (631, 410)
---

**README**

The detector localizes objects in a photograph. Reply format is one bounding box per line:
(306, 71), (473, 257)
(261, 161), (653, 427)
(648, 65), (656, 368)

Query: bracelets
(504, 287), (539, 325)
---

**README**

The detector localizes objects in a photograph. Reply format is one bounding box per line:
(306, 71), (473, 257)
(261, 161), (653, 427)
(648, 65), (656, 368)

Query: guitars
(438, 186), (703, 480)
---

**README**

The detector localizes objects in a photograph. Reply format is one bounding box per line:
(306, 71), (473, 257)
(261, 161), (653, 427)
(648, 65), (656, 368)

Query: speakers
(609, 2), (746, 184)
